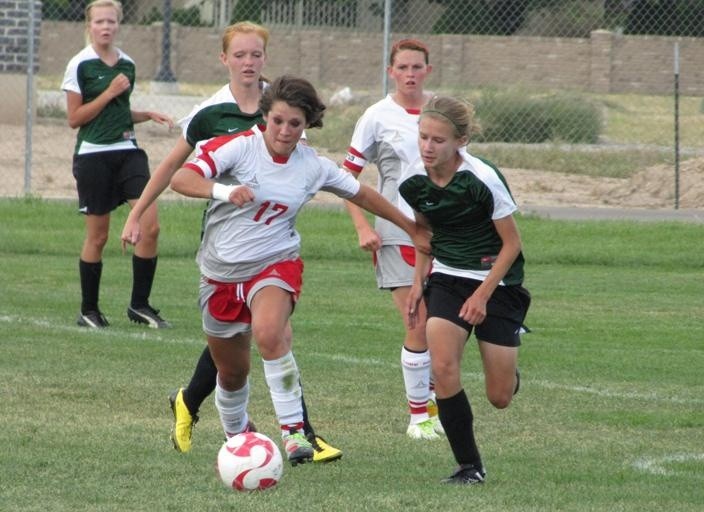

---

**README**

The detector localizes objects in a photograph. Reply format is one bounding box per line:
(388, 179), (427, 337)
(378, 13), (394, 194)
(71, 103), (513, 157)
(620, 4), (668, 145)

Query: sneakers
(78, 310), (110, 328)
(227, 368), (520, 485)
(127, 305), (172, 328)
(170, 388), (200, 454)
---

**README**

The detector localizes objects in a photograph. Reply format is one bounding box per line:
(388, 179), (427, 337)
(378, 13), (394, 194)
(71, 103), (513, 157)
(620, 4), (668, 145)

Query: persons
(403, 94), (530, 487)
(61, 0), (174, 328)
(342, 39), (446, 441)
(122, 20), (343, 465)
(170, 73), (433, 466)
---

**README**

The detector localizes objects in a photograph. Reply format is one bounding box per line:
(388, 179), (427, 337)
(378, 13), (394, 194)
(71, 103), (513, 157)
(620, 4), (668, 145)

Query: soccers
(218, 432), (283, 489)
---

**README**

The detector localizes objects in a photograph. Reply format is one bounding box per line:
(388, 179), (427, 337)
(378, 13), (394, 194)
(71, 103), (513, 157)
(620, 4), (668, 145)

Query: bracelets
(212, 183), (241, 202)
